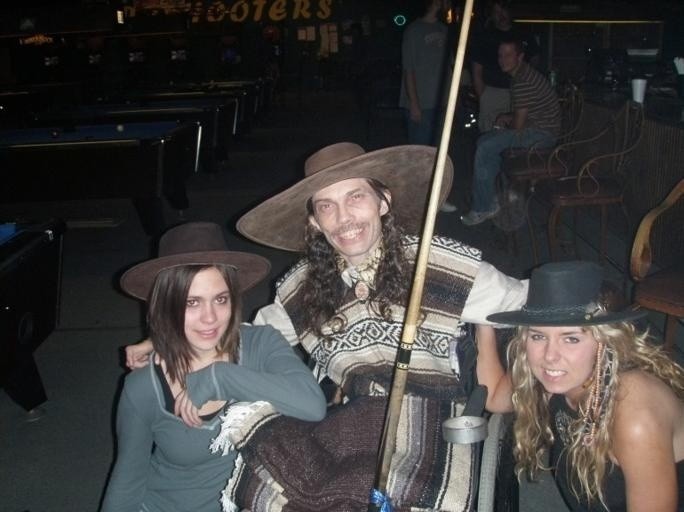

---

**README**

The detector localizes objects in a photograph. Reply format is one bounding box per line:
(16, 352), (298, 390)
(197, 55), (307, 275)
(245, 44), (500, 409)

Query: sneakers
(441, 188), (518, 225)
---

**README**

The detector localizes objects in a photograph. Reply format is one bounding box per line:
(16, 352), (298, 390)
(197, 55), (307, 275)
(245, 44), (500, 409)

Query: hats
(236, 141), (454, 250)
(486, 260), (650, 326)
(120, 221), (272, 301)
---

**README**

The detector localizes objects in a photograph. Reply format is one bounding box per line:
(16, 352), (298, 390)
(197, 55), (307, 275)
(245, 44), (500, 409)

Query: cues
(370, 1), (526, 508)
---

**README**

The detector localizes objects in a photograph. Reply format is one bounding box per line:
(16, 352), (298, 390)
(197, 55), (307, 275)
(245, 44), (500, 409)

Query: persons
(122, 174), (531, 510)
(96, 220), (327, 511)
(474, 258), (683, 510)
(471, 0), (542, 137)
(460, 37), (562, 229)
(394, 0), (458, 214)
